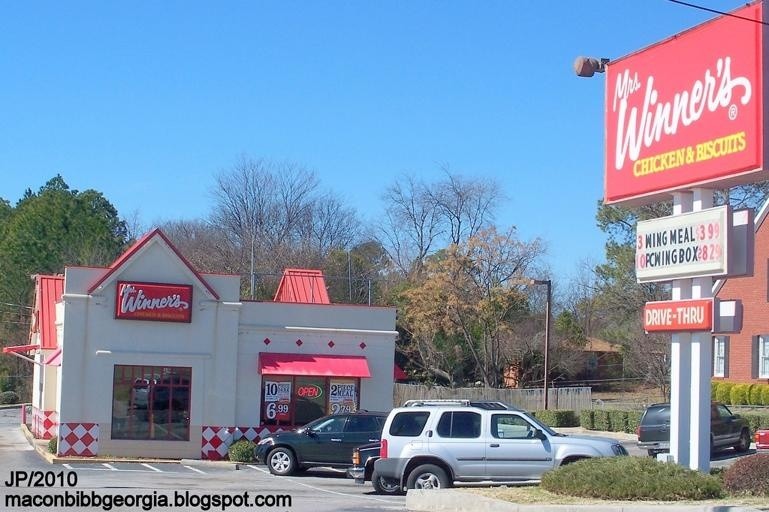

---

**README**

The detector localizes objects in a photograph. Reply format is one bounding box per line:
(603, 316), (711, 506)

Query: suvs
(636, 399), (751, 458)
(373, 398), (630, 492)
(253, 409), (388, 476)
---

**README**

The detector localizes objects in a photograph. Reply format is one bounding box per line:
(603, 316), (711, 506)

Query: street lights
(530, 279), (552, 408)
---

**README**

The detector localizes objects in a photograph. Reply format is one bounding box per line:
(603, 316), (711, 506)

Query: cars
(350, 439), (401, 493)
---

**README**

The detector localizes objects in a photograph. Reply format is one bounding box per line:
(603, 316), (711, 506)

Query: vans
(129, 373), (189, 409)
